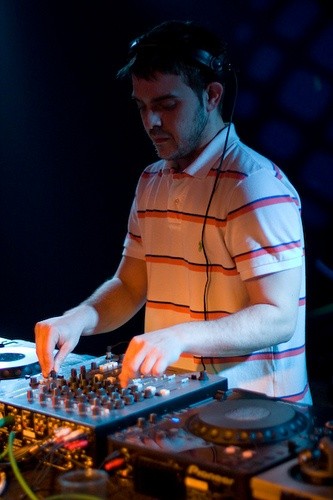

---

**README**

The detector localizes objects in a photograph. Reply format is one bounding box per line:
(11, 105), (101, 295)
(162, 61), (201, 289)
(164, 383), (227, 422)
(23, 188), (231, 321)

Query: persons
(35, 13), (315, 410)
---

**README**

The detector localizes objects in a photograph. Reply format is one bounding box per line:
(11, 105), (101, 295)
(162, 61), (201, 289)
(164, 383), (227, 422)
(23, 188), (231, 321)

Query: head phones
(127, 32), (232, 79)
